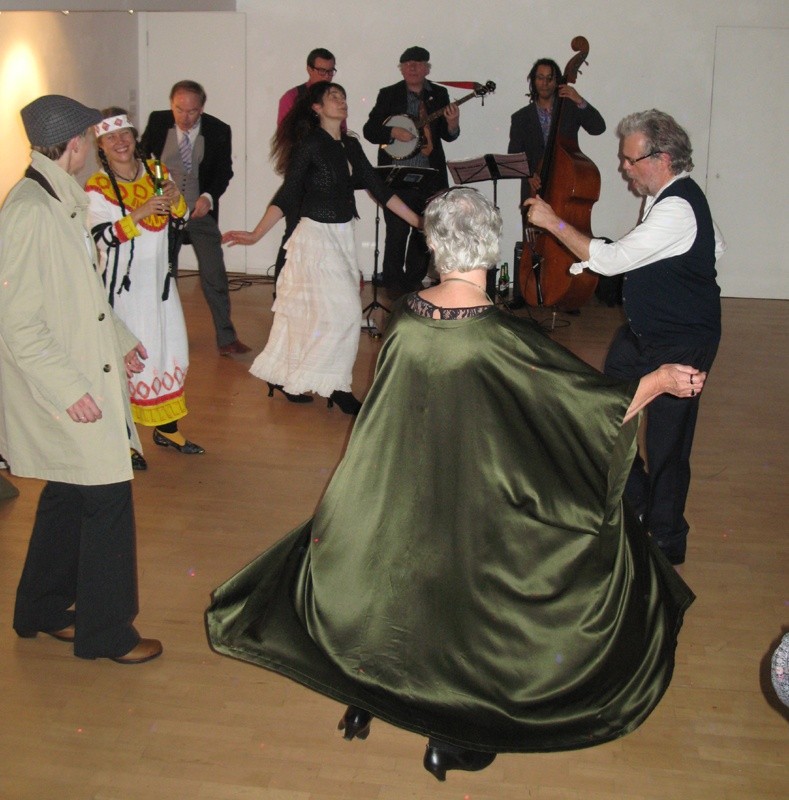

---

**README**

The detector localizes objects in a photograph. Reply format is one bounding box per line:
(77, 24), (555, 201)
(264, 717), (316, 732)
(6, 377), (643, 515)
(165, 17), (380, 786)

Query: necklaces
(444, 278), (492, 302)
(110, 160), (139, 182)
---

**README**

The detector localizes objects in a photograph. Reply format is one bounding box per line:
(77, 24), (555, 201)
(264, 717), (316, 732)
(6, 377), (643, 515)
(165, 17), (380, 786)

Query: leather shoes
(108, 636), (164, 665)
(13, 615), (77, 641)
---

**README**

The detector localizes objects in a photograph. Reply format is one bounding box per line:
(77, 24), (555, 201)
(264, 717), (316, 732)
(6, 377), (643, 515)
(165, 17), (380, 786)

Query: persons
(272, 48), (335, 310)
(363, 46), (460, 301)
(139, 80), (252, 356)
(83, 107), (205, 470)
(523, 108), (721, 564)
(0, 94), (161, 664)
(221, 81), (423, 415)
(508, 59), (606, 237)
(204, 186), (708, 783)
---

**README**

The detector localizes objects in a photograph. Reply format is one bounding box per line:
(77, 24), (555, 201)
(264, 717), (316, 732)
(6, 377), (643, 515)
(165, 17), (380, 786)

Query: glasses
(618, 149), (663, 166)
(313, 64), (339, 78)
(534, 74), (558, 84)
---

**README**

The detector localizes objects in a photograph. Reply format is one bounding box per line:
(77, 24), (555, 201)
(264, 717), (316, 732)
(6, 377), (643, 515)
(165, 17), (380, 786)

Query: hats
(18, 94), (104, 152)
(399, 45), (430, 63)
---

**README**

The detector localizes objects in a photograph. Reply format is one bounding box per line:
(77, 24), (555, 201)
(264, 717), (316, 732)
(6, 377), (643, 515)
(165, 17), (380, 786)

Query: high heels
(336, 703), (374, 741)
(128, 447), (147, 470)
(266, 379), (313, 403)
(422, 746), (497, 782)
(153, 428), (206, 455)
(325, 388), (362, 416)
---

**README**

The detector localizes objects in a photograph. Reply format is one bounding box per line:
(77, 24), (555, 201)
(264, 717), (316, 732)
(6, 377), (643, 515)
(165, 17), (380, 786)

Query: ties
(416, 95), (436, 157)
(177, 133), (194, 174)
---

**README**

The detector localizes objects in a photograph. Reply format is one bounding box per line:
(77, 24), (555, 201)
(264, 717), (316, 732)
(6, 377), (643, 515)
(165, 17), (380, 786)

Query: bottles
(155, 161), (168, 216)
(504, 263), (509, 294)
(499, 265), (506, 297)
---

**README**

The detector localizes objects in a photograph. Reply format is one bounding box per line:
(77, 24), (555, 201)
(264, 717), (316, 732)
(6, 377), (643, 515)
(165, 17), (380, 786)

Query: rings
(690, 375), (693, 384)
(527, 212), (531, 217)
(692, 390), (694, 395)
(128, 362), (131, 365)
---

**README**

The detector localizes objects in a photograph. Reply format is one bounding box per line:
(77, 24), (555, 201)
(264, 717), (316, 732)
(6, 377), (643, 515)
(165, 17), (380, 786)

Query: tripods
(349, 168), (436, 325)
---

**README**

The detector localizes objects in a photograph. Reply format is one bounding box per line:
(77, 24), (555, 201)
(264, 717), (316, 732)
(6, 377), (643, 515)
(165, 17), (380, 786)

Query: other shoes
(217, 339), (253, 356)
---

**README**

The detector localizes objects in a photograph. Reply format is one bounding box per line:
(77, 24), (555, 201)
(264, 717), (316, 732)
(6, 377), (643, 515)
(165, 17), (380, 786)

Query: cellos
(516, 33), (602, 334)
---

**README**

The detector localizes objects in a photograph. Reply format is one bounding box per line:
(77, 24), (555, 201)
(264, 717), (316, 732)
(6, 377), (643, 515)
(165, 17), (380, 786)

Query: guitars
(379, 79), (496, 161)
